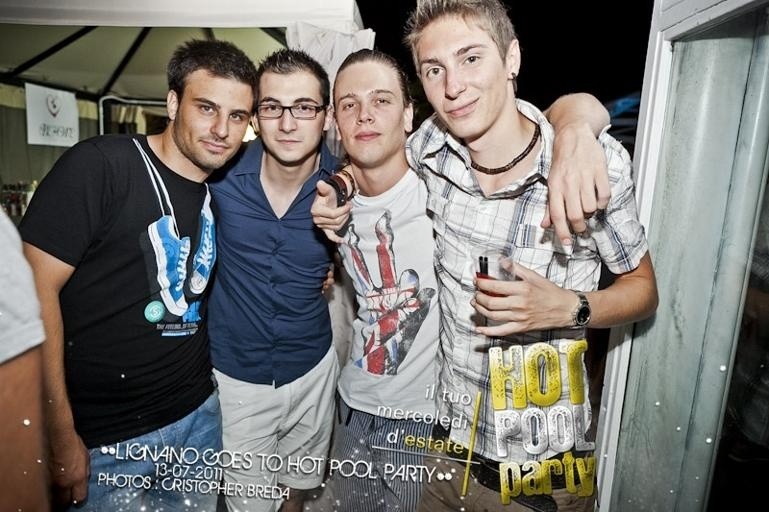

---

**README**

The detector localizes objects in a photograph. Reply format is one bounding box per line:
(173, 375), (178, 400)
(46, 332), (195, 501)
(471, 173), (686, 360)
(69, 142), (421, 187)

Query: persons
(310, 0), (659, 511)
(321, 49), (612, 511)
(17, 37), (336, 511)
(1, 207), (48, 512)
(207, 50), (346, 511)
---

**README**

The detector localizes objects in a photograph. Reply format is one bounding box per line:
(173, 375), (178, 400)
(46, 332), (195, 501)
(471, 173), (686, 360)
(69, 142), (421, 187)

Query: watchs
(566, 288), (591, 331)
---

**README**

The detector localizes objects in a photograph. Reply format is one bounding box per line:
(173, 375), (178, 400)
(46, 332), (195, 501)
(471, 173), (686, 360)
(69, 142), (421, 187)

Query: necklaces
(469, 124), (539, 174)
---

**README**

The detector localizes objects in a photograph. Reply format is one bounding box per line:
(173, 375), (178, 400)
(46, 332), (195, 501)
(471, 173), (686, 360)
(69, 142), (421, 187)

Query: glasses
(256, 103), (327, 120)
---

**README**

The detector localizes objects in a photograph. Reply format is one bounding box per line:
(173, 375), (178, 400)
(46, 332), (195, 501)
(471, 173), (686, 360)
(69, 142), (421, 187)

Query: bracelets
(326, 169), (356, 205)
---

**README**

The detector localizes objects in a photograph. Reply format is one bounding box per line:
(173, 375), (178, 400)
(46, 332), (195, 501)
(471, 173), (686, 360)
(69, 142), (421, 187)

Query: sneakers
(147, 215), (191, 316)
(190, 204), (218, 294)
